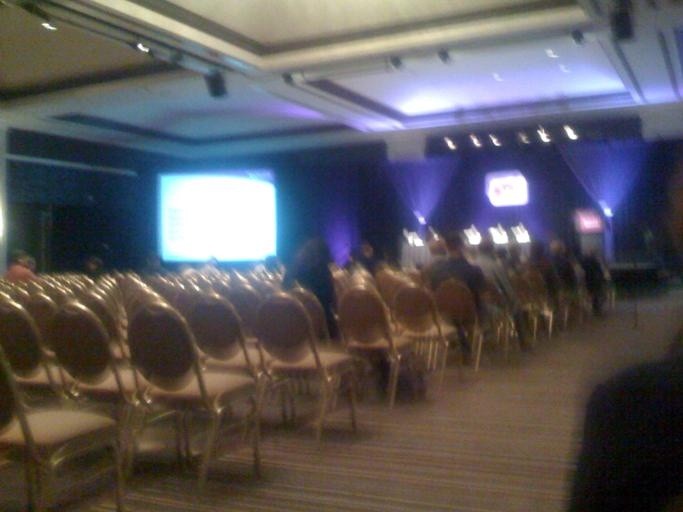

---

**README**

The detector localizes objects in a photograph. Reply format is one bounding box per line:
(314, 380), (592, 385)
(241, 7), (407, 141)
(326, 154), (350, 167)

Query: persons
(84, 255), (101, 272)
(429, 231), (608, 316)
(8, 247), (41, 282)
(346, 239), (377, 271)
(282, 244), (340, 340)
(145, 247), (160, 270)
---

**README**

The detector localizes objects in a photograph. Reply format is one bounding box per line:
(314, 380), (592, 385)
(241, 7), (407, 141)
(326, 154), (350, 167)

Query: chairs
(2, 264), (358, 510)
(338, 266), (617, 408)
